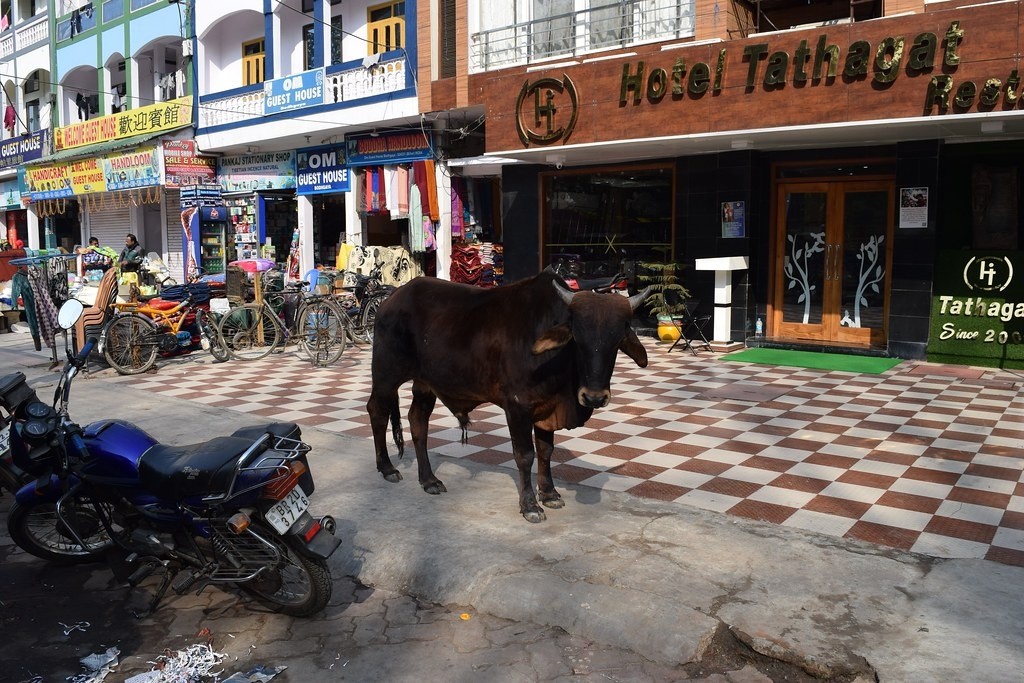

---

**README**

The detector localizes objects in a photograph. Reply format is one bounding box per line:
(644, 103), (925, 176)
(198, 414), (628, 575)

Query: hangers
(14, 255), (65, 273)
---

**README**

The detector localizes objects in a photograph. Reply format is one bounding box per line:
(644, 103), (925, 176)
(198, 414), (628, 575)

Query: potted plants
(641, 262), (691, 342)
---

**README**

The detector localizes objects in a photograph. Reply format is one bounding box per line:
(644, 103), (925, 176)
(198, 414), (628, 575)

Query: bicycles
(97, 260), (399, 376)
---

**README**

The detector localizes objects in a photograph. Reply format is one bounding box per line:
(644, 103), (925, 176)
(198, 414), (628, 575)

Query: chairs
(76, 267), (117, 369)
(662, 290), (715, 356)
(301, 268), (329, 340)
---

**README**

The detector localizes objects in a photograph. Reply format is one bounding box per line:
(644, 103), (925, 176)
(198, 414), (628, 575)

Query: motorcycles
(0, 297), (344, 621)
(545, 256), (630, 299)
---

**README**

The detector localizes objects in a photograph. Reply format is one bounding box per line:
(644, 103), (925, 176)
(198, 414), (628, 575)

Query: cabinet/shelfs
(223, 194), (265, 262)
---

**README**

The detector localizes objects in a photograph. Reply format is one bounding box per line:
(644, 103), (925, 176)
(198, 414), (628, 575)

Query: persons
(88, 237), (100, 249)
(119, 233), (144, 276)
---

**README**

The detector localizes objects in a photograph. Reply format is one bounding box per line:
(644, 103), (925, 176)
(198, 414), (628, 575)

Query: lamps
(246, 145), (252, 154)
(371, 126), (379, 137)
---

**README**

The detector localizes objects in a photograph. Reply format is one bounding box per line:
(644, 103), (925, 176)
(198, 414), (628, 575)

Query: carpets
(718, 347), (905, 376)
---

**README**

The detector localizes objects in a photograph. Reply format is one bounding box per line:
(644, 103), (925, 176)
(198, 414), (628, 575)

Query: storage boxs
(261, 237), (275, 262)
(315, 275), (349, 296)
(225, 196), (256, 257)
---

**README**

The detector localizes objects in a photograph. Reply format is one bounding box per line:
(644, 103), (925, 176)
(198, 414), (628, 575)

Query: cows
(364, 263), (650, 524)
